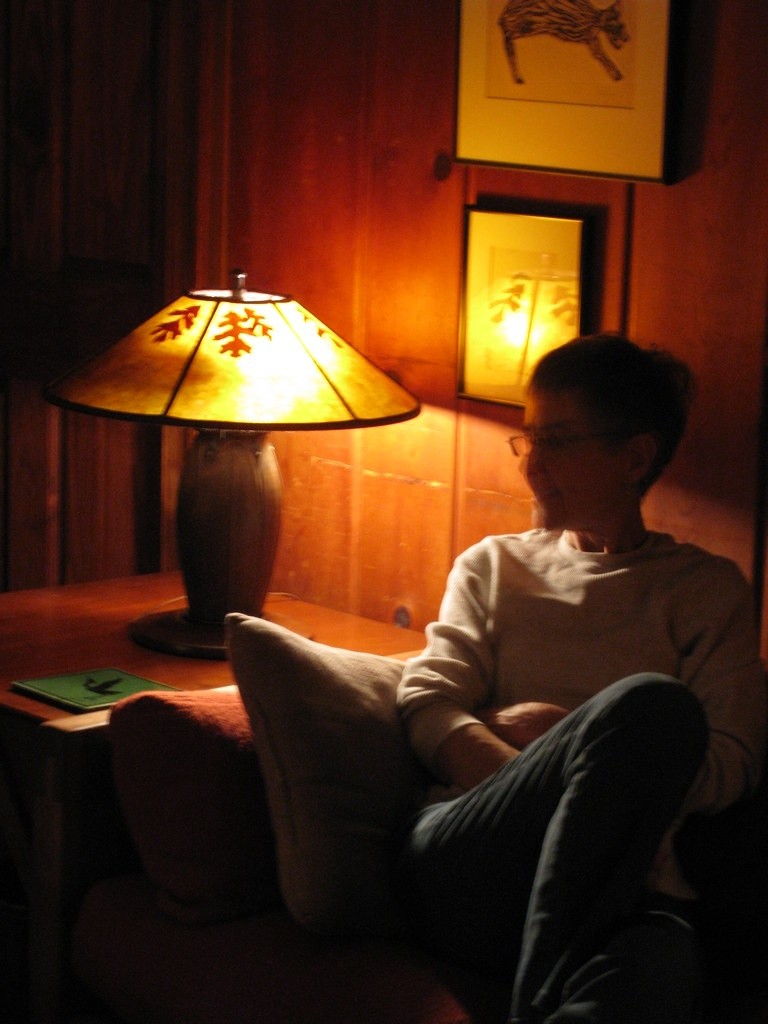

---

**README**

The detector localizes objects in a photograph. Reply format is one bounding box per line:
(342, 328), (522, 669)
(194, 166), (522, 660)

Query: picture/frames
(447, 0), (687, 185)
(455, 203), (594, 412)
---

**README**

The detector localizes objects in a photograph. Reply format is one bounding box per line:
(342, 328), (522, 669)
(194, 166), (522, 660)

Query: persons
(396, 329), (768, 1023)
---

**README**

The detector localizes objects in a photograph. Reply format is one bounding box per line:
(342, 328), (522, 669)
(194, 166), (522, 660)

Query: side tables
(0, 567), (426, 1024)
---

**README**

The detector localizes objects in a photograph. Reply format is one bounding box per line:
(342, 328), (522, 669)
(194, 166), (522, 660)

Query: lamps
(39, 270), (420, 661)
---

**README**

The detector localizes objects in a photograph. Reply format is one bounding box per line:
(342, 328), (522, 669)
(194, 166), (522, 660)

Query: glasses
(509, 430), (622, 456)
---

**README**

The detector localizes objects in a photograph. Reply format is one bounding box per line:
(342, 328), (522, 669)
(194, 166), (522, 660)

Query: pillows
(222, 611), (432, 939)
(109, 685), (280, 927)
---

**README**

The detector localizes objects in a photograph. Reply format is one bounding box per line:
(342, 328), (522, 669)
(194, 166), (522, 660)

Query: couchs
(71, 872), (482, 1024)
